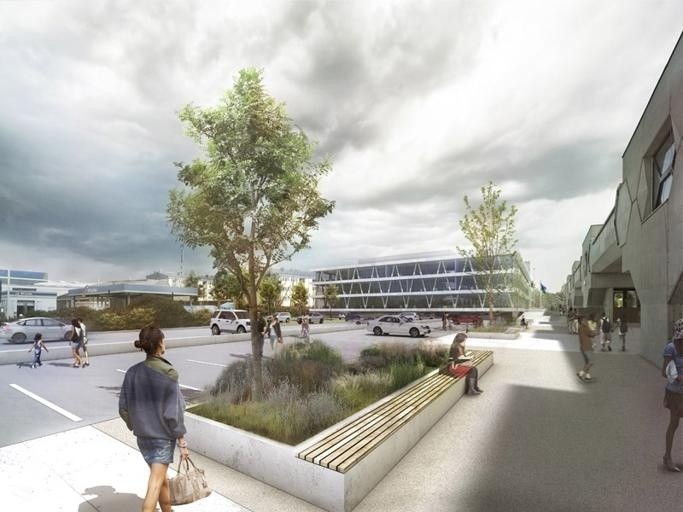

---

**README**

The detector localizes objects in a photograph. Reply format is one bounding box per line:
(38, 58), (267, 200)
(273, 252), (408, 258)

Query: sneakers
(577, 371), (596, 383)
(74, 360), (89, 368)
(601, 345), (611, 352)
(32, 363), (42, 368)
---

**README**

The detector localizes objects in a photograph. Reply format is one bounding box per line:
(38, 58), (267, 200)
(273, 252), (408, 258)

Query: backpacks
(601, 318), (609, 333)
(620, 321), (628, 333)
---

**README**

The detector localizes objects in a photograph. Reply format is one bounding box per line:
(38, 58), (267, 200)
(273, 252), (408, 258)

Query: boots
(467, 377), (483, 395)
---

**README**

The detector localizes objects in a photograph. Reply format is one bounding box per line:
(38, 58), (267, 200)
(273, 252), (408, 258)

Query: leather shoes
(663, 456), (680, 472)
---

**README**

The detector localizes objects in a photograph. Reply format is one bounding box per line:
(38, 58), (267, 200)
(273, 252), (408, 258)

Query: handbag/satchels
(169, 457), (211, 505)
(71, 333), (78, 341)
(448, 364), (469, 380)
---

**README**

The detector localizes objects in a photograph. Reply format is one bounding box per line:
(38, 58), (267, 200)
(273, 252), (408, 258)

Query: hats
(672, 319), (683, 339)
